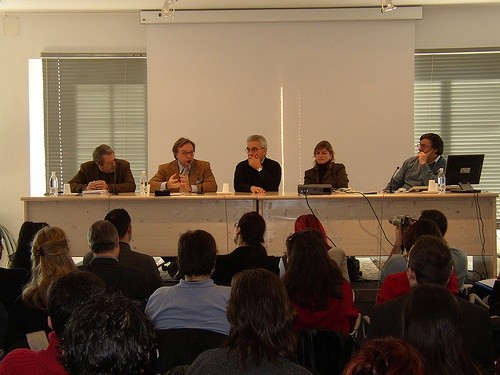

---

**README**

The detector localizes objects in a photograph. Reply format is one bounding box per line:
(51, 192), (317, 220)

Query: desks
(22, 191), (260, 257)
(258, 188), (500, 280)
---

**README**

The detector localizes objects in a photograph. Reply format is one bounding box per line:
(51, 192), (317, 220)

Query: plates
(423, 191), (440, 194)
(216, 192), (233, 195)
(63, 193), (78, 195)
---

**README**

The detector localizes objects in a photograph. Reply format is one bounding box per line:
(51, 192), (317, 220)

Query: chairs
(217, 253), (281, 288)
(151, 325), (229, 372)
(5, 303), (51, 346)
(0, 266), (31, 321)
(292, 326), (361, 375)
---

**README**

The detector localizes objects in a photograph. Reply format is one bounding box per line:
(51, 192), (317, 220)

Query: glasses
(245, 147), (264, 153)
(178, 149), (195, 156)
(234, 223), (241, 229)
(403, 253), (415, 269)
(417, 144), (436, 149)
(288, 230), (312, 247)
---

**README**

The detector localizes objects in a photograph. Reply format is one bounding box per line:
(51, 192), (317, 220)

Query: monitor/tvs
(445, 155), (485, 193)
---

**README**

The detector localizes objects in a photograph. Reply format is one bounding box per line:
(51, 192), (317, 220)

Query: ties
(180, 167), (189, 192)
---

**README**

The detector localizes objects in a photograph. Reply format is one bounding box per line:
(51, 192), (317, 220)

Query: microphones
(112, 161), (116, 195)
(187, 160), (204, 194)
(389, 167), (399, 193)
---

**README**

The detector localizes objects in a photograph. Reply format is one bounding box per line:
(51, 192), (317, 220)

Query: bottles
(49, 171), (58, 196)
(140, 171), (149, 196)
(437, 168), (445, 193)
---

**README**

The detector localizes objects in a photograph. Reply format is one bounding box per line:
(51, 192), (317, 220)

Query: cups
(64, 184), (70, 193)
(148, 185), (150, 194)
(429, 180), (435, 190)
(223, 184), (229, 192)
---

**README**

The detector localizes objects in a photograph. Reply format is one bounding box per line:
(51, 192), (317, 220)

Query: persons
(0, 208), (500, 375)
(68, 144), (136, 194)
(304, 140), (350, 189)
(149, 137), (218, 193)
(382, 133), (447, 193)
(233, 135), (282, 193)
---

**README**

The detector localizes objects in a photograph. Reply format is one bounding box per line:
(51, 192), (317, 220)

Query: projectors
(298, 184), (333, 195)
